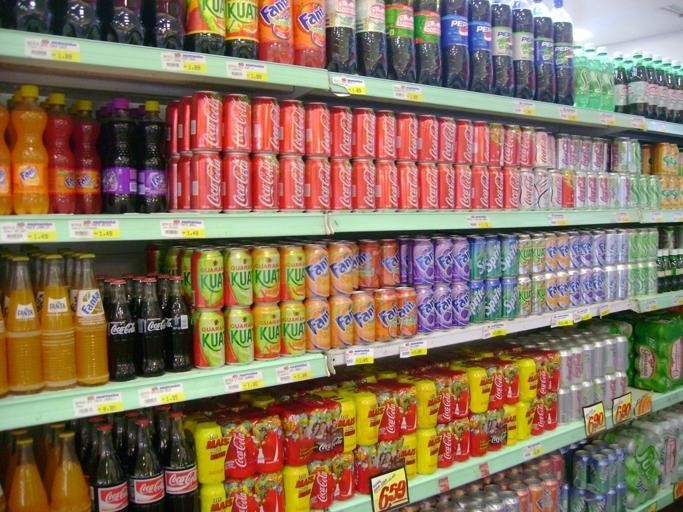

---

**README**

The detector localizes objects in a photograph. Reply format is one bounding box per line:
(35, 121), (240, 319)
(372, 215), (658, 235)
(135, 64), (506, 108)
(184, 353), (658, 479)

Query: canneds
(162, 312), (682, 512)
(146, 225), (683, 372)
(166, 92), (683, 213)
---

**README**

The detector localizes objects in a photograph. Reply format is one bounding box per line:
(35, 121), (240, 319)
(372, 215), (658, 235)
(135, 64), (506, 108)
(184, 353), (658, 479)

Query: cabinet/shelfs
(0, 28), (683, 512)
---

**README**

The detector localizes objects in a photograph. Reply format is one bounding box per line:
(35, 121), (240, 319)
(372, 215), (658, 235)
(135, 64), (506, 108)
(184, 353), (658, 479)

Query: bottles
(0, 1), (575, 104)
(657, 246), (683, 292)
(0, 81), (169, 214)
(574, 44), (682, 125)
(6, 406), (199, 511)
(0, 245), (192, 395)
